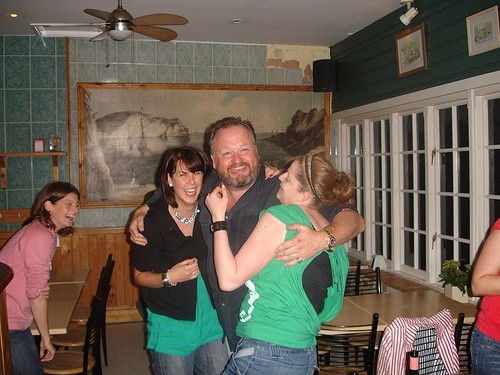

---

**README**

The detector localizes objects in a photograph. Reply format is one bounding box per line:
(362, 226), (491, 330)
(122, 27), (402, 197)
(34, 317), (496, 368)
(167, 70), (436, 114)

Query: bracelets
(167, 269), (177, 286)
(211, 221), (228, 232)
(161, 272), (173, 288)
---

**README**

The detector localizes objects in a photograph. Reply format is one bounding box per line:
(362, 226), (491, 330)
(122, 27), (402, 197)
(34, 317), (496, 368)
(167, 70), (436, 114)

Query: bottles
(405, 350), (419, 375)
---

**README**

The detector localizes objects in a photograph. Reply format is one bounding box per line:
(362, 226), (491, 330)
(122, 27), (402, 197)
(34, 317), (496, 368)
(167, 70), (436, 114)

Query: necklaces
(174, 201), (198, 224)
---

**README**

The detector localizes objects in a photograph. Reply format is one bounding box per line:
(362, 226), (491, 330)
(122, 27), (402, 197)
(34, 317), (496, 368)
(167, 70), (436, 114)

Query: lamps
(399, 0), (418, 25)
(105, 22), (134, 68)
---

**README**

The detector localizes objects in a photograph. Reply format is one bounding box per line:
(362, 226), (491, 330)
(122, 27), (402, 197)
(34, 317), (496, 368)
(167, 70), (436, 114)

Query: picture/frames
(466, 6), (500, 57)
(394, 23), (428, 78)
(77, 82), (330, 209)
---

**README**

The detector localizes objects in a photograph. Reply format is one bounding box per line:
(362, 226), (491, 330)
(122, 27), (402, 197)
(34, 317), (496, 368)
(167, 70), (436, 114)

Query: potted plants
(438, 259), (473, 304)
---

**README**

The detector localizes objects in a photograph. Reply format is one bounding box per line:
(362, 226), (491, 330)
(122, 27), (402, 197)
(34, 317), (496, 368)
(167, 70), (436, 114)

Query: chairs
(313, 261), (473, 375)
(42, 254), (115, 375)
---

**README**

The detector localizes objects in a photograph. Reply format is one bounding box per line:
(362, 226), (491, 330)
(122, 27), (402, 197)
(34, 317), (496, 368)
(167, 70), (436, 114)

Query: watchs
(323, 228), (336, 249)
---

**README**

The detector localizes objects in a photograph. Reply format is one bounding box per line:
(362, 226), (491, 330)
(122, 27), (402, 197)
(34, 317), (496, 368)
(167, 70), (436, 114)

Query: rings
(297, 258), (302, 263)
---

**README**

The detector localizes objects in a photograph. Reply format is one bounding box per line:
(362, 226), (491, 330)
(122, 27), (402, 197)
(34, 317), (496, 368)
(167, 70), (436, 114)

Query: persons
(204, 155), (355, 375)
(129, 116), (365, 353)
(133, 145), (229, 375)
(470, 218), (500, 375)
(0, 181), (80, 375)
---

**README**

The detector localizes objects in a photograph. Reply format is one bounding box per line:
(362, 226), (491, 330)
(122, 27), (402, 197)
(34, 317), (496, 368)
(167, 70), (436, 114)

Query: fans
(83, 0), (189, 42)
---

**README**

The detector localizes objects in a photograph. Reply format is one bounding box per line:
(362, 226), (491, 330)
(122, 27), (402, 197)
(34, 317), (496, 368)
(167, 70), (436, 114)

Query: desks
(46, 267), (91, 284)
(346, 292), (478, 325)
(29, 283), (84, 335)
(319, 298), (389, 335)
